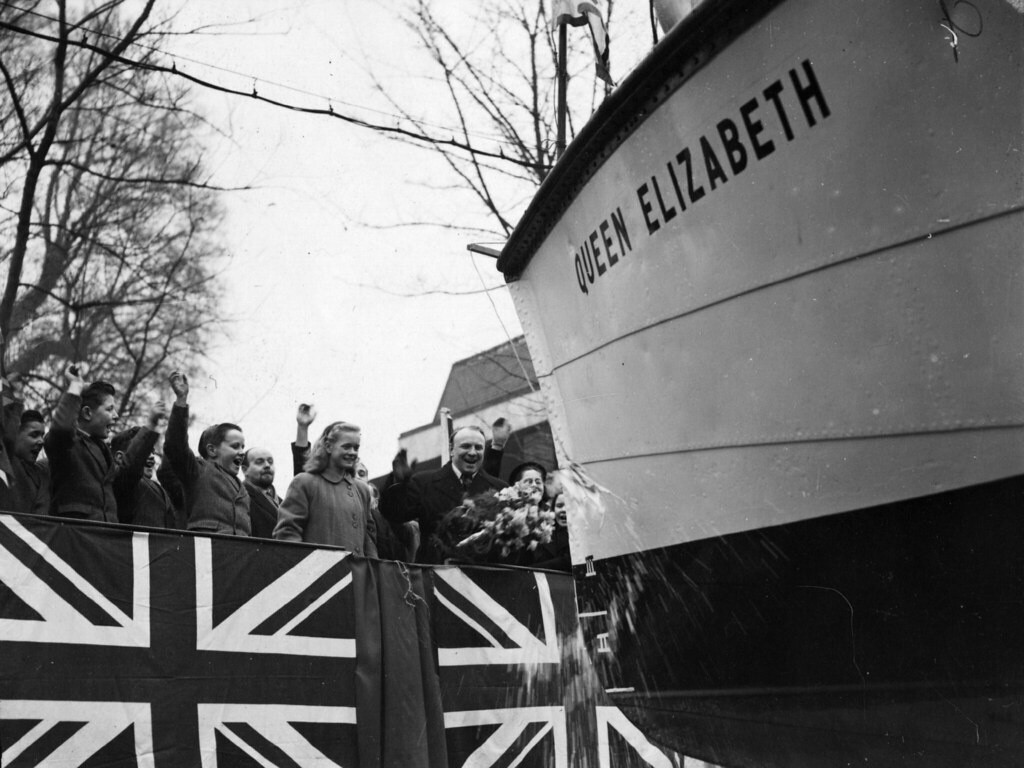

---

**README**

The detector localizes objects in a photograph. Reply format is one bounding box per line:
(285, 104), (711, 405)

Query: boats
(495, 0), (1021, 768)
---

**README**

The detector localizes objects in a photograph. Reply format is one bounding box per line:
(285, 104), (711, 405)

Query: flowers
(454, 481), (555, 557)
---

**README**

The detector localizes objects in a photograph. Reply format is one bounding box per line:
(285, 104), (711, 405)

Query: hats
(507, 462), (548, 485)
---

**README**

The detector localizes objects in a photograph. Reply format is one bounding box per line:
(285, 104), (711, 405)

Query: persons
(0, 364), (573, 578)
